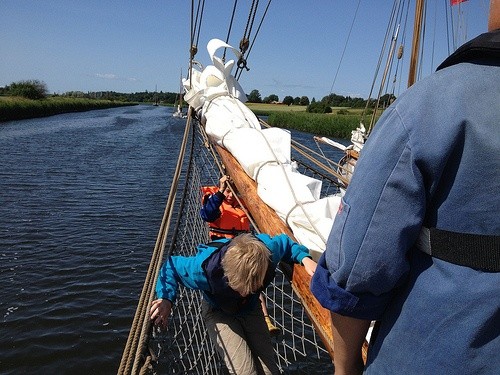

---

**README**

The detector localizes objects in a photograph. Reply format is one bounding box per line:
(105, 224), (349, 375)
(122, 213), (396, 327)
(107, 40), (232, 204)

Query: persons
(201, 175), (282, 337)
(308, 30), (500, 375)
(149, 231), (318, 375)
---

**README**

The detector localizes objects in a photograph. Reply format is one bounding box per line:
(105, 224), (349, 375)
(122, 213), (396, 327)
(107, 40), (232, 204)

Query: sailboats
(153, 84), (159, 106)
(172, 66), (188, 118)
